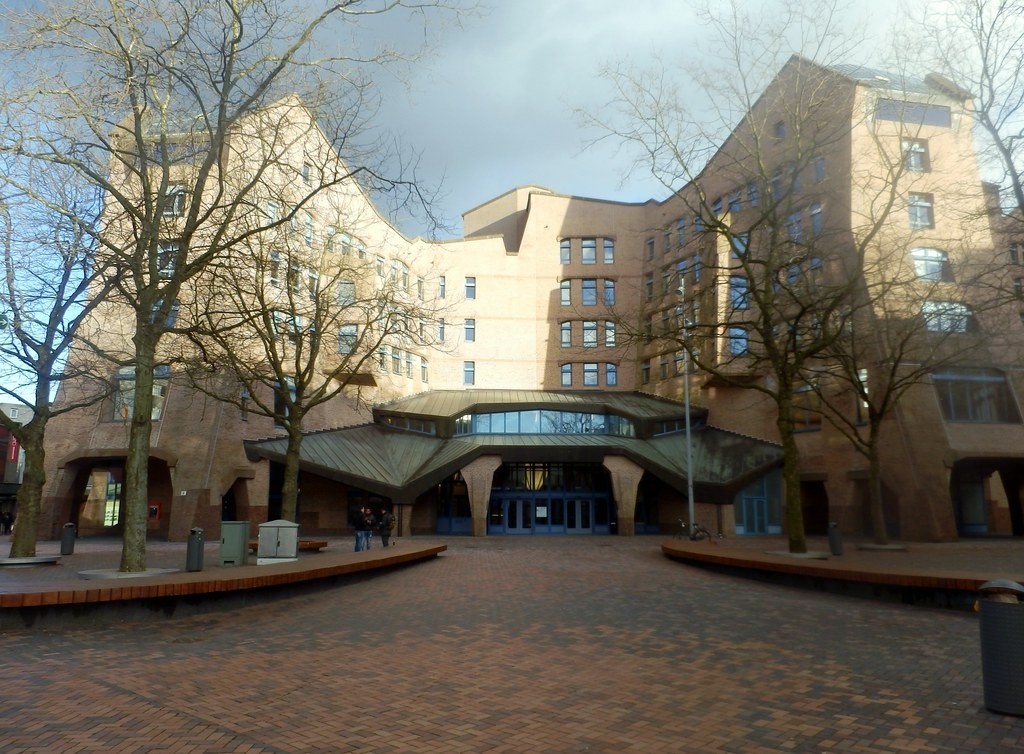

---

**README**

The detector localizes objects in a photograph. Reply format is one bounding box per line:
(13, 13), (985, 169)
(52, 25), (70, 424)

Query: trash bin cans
(60, 523), (76, 555)
(185, 527), (205, 571)
(976, 580), (1023, 717)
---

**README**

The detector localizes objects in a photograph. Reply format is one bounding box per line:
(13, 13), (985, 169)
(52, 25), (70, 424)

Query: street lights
(676, 277), (697, 538)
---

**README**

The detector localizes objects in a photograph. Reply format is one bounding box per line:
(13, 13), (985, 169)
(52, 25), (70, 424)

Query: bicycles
(673, 518), (712, 543)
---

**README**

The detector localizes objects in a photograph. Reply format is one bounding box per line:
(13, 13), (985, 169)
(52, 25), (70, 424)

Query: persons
(377, 509), (391, 546)
(353, 506), (369, 552)
(362, 508), (376, 551)
(2, 515), (12, 535)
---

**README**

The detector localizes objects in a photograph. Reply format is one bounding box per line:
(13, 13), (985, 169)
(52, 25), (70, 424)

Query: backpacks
(386, 514), (395, 530)
